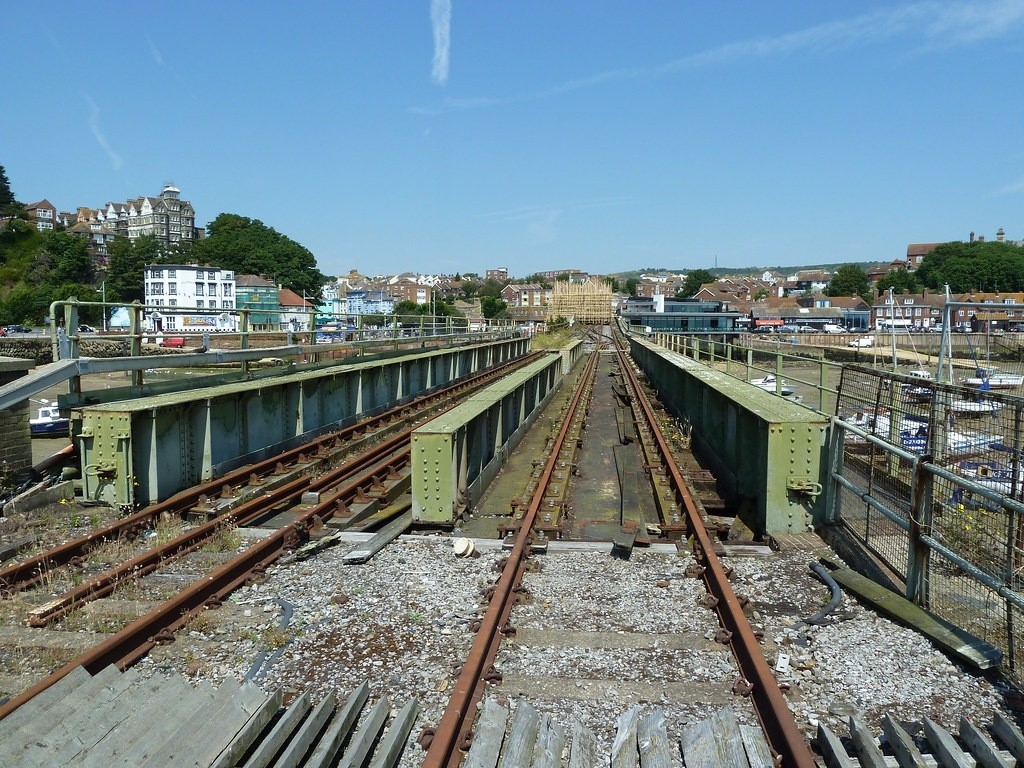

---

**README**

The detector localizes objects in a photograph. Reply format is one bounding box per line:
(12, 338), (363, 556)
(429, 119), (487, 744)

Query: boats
(30, 398), (71, 438)
(751, 279), (1023, 513)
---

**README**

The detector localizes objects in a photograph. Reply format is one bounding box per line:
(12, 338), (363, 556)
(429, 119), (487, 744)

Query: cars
(801, 326), (819, 333)
(850, 327), (870, 333)
(6, 324), (32, 333)
(778, 324), (796, 332)
(80, 324), (94, 333)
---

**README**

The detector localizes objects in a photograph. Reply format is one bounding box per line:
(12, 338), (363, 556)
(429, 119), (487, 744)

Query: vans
(753, 325), (772, 334)
(823, 324), (846, 334)
(849, 339), (872, 347)
(159, 337), (186, 349)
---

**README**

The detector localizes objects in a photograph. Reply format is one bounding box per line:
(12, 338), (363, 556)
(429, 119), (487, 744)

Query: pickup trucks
(928, 323), (944, 332)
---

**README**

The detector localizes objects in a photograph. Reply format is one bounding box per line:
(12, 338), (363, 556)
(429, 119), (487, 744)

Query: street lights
(95, 281), (106, 330)
